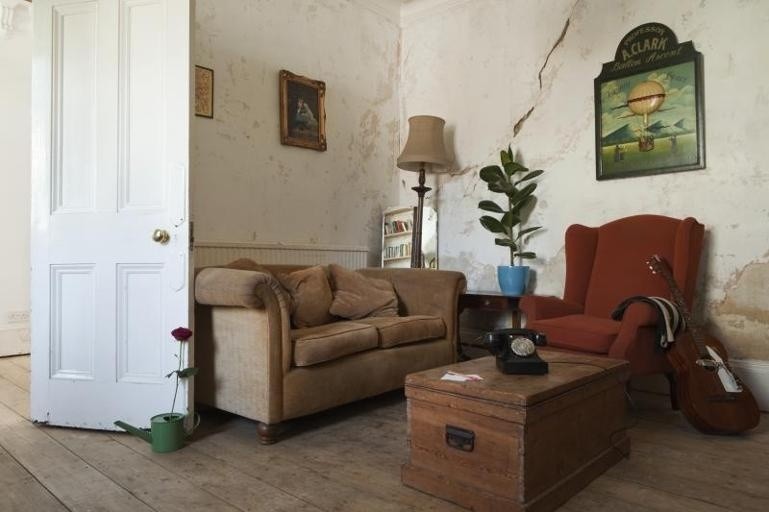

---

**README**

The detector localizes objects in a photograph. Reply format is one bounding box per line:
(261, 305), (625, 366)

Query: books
(384, 220), (414, 235)
(384, 242), (412, 259)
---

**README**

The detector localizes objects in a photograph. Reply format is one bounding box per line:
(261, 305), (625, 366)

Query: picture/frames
(593, 23), (705, 181)
(278, 69), (327, 152)
(195, 66), (213, 118)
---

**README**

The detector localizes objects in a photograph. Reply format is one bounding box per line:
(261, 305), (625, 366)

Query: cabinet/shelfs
(381, 206), (438, 272)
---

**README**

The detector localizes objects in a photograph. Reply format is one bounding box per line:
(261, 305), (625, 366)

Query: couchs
(192, 256), (468, 446)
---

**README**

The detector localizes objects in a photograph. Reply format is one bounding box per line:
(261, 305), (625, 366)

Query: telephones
(484, 328), (549, 375)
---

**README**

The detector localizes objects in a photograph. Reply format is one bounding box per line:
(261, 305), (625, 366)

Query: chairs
(516, 214), (706, 411)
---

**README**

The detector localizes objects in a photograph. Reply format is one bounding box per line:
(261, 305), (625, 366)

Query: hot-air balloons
(625, 80), (666, 152)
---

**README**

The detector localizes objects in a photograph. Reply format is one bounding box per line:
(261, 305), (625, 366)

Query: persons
(293, 97), (318, 131)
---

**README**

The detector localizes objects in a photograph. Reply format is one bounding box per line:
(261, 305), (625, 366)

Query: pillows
(222, 256), (400, 327)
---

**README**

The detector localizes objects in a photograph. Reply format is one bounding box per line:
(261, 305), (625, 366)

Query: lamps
(395, 115), (451, 267)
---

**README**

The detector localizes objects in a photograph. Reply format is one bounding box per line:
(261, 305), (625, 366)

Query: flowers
(165, 325), (200, 422)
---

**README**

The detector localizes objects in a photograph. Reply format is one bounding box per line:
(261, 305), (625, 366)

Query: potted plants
(474, 145), (545, 297)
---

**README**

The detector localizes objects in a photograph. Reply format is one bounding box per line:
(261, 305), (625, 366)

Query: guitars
(646, 256), (759, 433)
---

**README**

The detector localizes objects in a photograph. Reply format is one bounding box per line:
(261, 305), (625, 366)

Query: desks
(457, 289), (533, 361)
